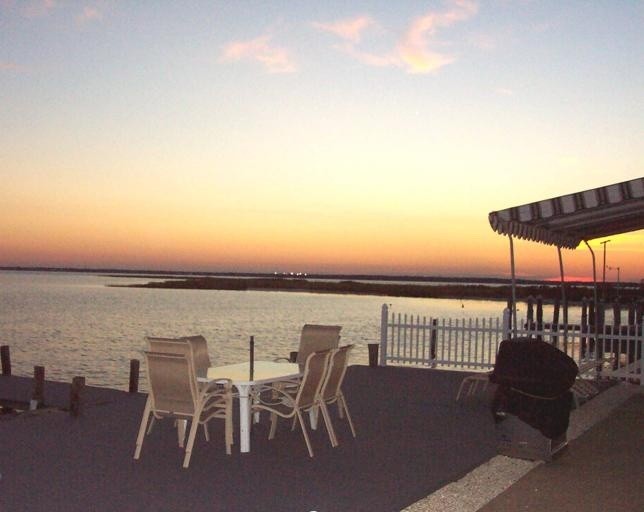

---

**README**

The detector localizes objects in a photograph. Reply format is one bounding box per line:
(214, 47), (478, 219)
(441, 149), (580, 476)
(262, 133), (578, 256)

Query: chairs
(455, 360), (605, 409)
(133, 325), (356, 467)
